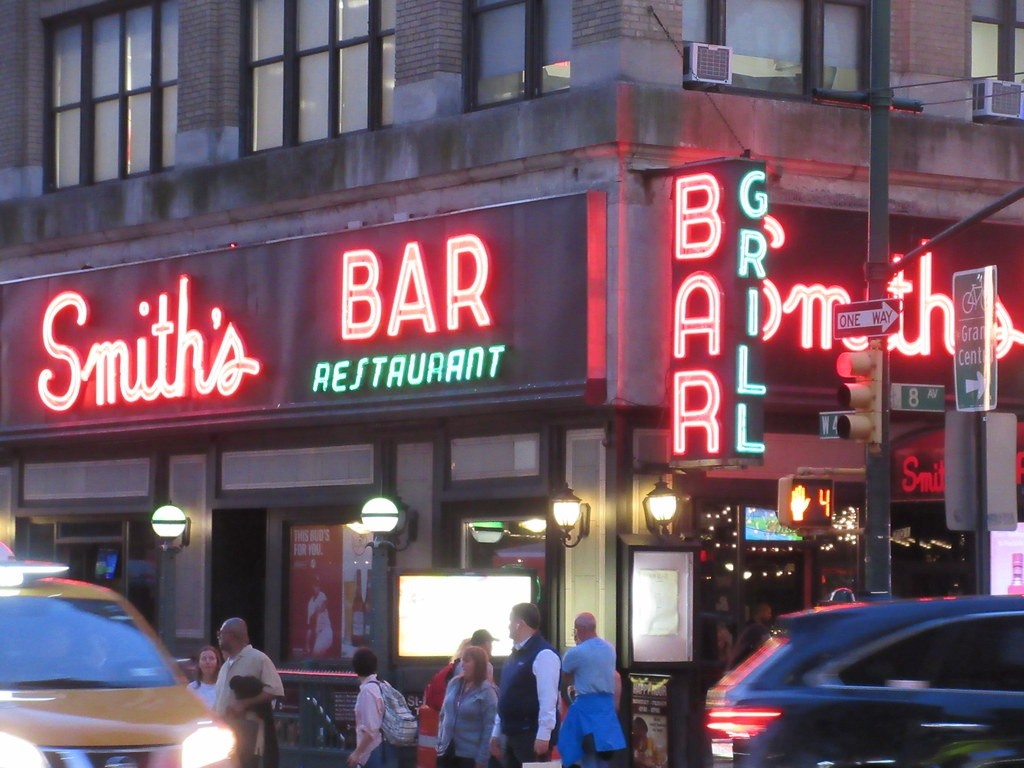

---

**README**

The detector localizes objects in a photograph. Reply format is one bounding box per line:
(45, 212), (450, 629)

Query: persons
(556, 613), (626, 768)
(187, 644), (222, 708)
(728, 604), (772, 672)
(433, 629), (499, 768)
(345, 648), (401, 768)
(212, 618), (283, 768)
(488, 603), (561, 768)
(303, 575), (333, 657)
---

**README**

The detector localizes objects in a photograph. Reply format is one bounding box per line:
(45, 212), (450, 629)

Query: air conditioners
(682, 42), (732, 84)
(968, 81), (1023, 122)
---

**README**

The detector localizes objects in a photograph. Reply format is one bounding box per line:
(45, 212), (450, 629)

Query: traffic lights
(834, 347), (886, 446)
(776, 474), (835, 531)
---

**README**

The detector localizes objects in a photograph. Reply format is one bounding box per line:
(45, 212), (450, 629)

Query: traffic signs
(951, 263), (1000, 413)
(831, 296), (902, 341)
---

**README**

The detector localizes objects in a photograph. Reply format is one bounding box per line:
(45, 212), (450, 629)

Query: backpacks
(364, 678), (418, 747)
(423, 658), (462, 711)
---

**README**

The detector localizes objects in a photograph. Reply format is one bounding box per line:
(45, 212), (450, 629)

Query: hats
(471, 629), (499, 645)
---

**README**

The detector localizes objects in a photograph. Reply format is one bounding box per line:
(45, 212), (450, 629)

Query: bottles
(365, 570), (375, 645)
(351, 569), (364, 646)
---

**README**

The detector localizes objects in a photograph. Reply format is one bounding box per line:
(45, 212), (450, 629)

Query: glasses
(216, 630), (237, 636)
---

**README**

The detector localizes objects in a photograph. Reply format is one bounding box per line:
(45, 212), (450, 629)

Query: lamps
(469, 521), (504, 546)
(642, 474), (688, 536)
(349, 494), (406, 551)
(152, 505), (192, 556)
(553, 482), (594, 549)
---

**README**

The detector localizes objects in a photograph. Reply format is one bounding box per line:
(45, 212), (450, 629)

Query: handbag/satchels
(222, 710), (258, 768)
(523, 753), (562, 768)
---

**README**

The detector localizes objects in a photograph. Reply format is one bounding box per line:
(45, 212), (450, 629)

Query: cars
(0, 539), (236, 768)
(702, 593), (1024, 768)
